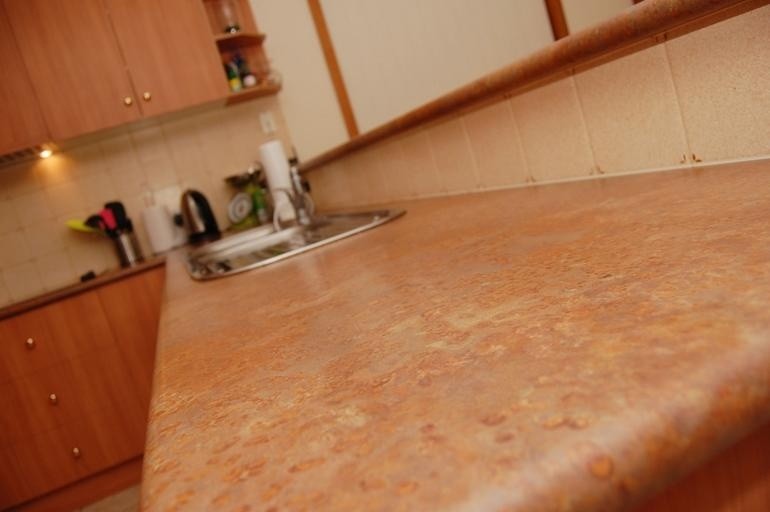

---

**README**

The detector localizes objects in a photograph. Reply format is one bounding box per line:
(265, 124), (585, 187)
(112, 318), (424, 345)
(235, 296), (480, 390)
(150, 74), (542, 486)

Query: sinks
(180, 206), (407, 279)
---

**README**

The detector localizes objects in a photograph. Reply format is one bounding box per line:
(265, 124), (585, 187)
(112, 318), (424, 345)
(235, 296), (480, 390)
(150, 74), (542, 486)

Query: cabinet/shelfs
(203, 0), (281, 107)
(0, 0), (233, 150)
(0, 265), (166, 512)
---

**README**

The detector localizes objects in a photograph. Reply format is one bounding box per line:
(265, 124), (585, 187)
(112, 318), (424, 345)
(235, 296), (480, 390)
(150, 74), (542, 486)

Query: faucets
(270, 164), (322, 231)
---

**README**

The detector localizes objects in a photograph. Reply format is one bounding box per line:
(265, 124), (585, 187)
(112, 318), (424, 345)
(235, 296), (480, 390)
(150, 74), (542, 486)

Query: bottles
(111, 221), (144, 265)
(224, 50), (255, 91)
(254, 190), (267, 224)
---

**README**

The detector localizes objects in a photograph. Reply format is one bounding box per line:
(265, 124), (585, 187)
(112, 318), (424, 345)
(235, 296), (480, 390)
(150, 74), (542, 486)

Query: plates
(227, 193), (253, 224)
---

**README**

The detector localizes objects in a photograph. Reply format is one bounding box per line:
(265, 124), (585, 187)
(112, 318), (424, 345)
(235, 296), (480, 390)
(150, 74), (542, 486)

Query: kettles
(175, 190), (220, 244)
